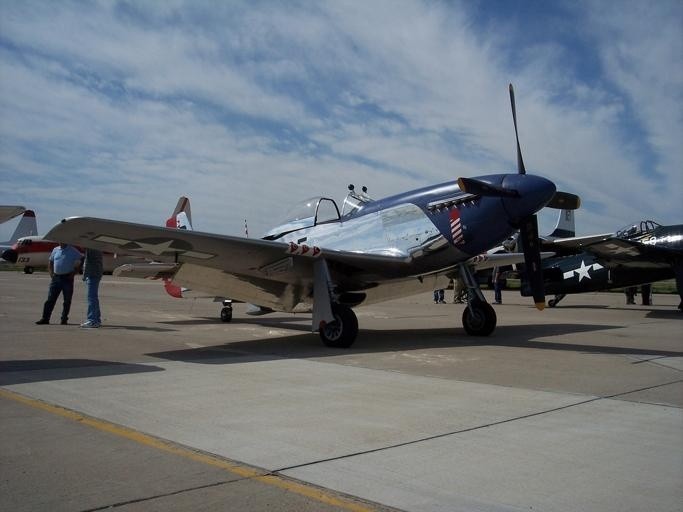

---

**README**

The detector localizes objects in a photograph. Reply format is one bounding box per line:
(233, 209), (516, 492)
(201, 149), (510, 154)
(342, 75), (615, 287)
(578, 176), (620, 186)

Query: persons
(490, 263), (502, 305)
(642, 284), (652, 304)
(624, 286), (637, 305)
(78, 249), (102, 329)
(433, 289), (444, 304)
(33, 243), (85, 327)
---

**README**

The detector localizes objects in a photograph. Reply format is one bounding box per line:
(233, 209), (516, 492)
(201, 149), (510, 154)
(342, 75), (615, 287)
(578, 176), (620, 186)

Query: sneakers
(60, 320), (67, 324)
(35, 319), (50, 325)
(80, 321), (102, 328)
(492, 302), (502, 304)
(434, 301), (446, 304)
